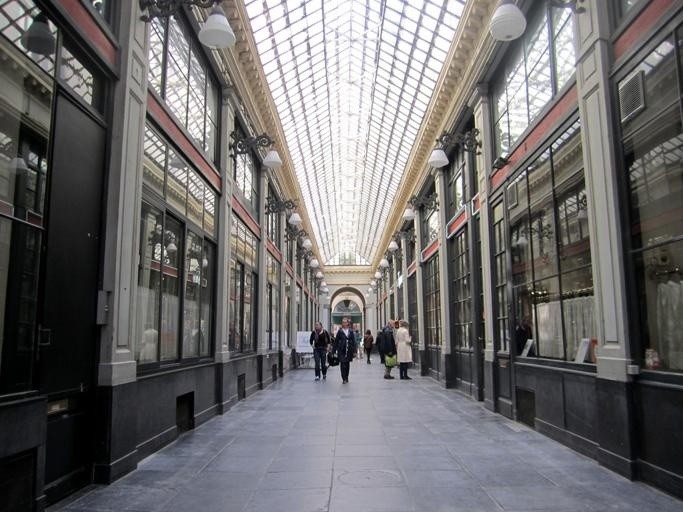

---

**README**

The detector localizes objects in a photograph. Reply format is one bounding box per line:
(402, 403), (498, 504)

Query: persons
(310, 321), (331, 380)
(375, 321), (412, 379)
(364, 330), (373, 364)
(331, 317), (363, 384)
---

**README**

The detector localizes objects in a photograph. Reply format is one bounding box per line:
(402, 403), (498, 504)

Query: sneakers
(400, 376), (411, 379)
(384, 375), (394, 379)
(343, 378), (349, 384)
(315, 375), (326, 380)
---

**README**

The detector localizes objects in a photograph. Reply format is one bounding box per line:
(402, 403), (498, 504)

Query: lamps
(266, 195), (330, 294)
(139, 3), (235, 49)
(364, 192), (439, 299)
(229, 132), (281, 168)
(428, 130), (482, 168)
(490, 0), (586, 40)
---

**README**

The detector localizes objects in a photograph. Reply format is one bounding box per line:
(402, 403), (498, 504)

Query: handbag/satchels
(384, 354), (396, 367)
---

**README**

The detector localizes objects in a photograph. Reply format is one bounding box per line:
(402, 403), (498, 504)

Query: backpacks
(328, 352), (339, 366)
